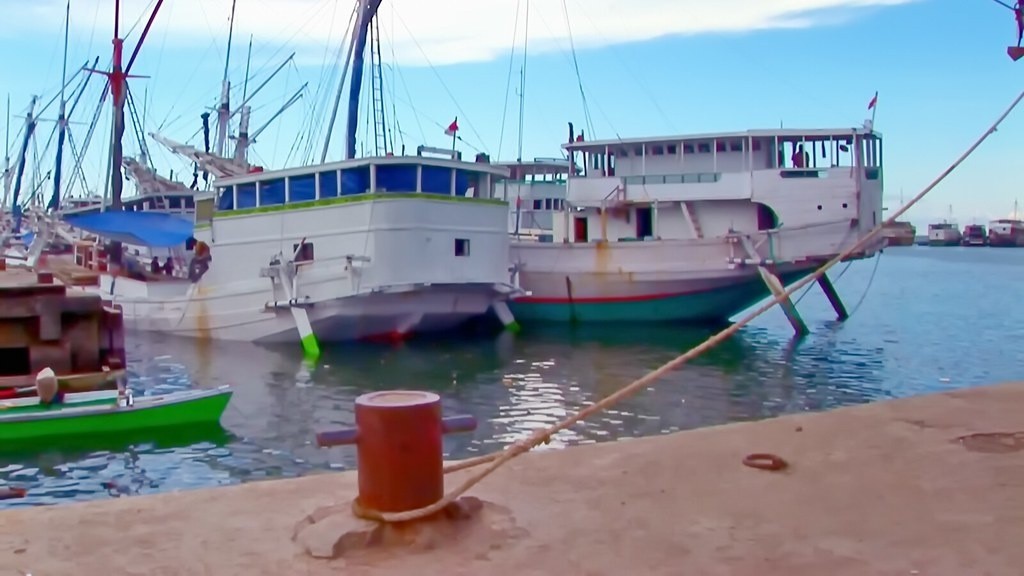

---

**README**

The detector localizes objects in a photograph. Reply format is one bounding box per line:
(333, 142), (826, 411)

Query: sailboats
(0, 1), (530, 357)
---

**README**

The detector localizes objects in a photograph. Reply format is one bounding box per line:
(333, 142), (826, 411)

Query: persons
(162, 257), (174, 276)
(151, 256), (163, 274)
(794, 144), (809, 169)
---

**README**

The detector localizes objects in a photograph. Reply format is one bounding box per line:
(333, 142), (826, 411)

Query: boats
(963, 224), (987, 246)
(882, 221), (916, 246)
(0, 382), (235, 457)
(493, 0), (889, 322)
(988, 219), (1024, 246)
(0, 251), (124, 404)
(928, 223), (962, 245)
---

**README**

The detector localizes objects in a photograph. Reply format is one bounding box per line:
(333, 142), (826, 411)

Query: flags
(868, 95), (878, 109)
(445, 122), (459, 133)
